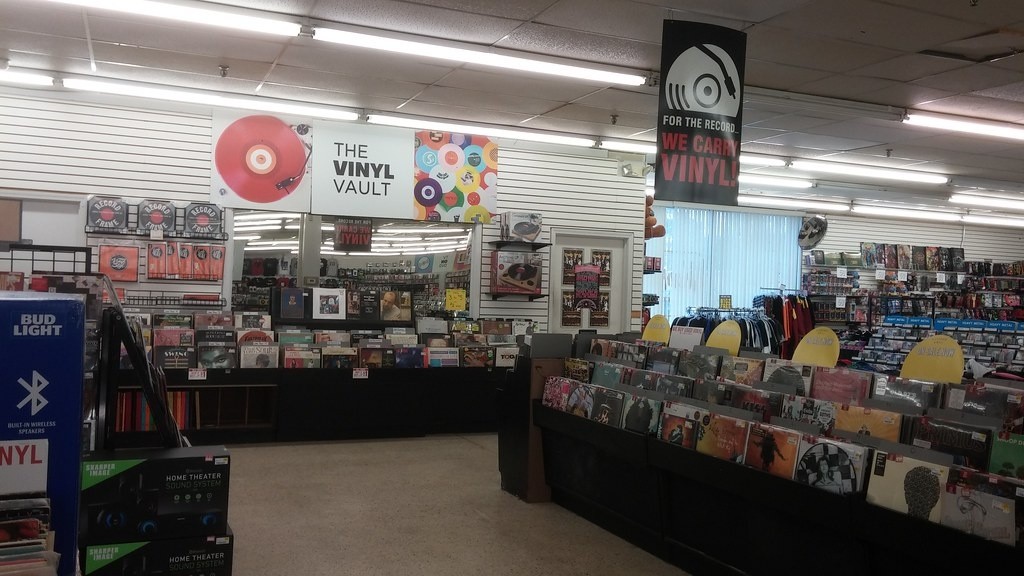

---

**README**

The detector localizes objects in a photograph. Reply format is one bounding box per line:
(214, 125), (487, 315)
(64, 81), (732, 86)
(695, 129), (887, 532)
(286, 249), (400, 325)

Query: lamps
(0, 0), (1024, 231)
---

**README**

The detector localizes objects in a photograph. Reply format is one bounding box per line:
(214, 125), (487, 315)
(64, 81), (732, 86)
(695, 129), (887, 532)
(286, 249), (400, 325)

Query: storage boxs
(500, 211), (543, 243)
(77, 444), (231, 548)
(79, 523), (234, 576)
(653, 257), (662, 271)
(644, 256), (653, 270)
(490, 251), (543, 293)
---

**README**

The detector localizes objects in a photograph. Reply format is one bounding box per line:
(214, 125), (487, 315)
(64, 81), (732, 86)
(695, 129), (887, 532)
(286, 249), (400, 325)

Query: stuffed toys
(644, 194), (667, 239)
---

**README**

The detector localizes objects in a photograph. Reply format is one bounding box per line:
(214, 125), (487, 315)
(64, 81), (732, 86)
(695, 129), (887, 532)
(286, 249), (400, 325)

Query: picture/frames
(313, 287), (347, 320)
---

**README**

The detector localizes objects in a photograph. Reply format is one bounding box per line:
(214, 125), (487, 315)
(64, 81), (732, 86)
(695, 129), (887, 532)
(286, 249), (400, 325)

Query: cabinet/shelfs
(806, 263), (1024, 333)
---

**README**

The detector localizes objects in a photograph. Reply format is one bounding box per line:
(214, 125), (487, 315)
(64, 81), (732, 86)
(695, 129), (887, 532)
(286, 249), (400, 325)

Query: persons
(564, 336), (955, 529)
(319, 297), (340, 314)
(255, 354), (379, 368)
(381, 292), (402, 320)
(452, 319), (538, 367)
(126, 315), (267, 369)
(285, 293), (302, 317)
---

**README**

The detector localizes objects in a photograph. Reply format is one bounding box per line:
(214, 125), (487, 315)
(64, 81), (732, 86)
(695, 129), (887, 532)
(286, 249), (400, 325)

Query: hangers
(757, 287), (807, 298)
(684, 306), (770, 321)
